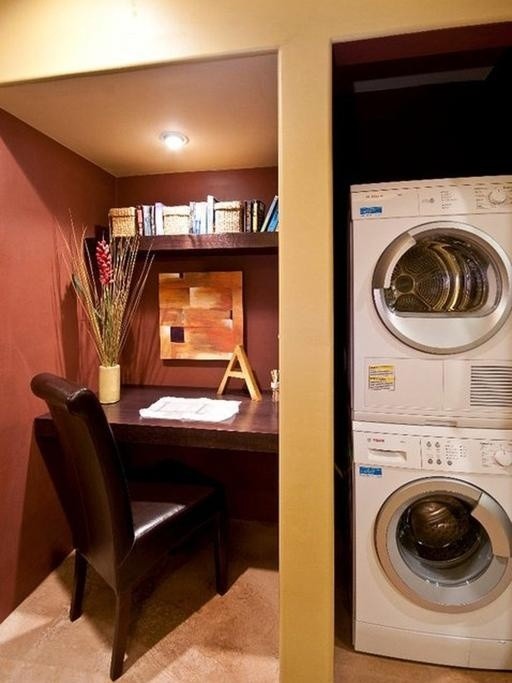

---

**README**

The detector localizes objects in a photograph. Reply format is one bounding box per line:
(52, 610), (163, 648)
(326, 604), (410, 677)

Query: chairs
(27, 372), (227, 683)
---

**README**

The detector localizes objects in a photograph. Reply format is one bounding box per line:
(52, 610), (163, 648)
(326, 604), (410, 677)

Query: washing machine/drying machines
(350, 420), (512, 672)
(347, 171), (512, 420)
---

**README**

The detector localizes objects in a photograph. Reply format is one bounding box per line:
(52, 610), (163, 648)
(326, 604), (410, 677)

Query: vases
(98, 365), (122, 402)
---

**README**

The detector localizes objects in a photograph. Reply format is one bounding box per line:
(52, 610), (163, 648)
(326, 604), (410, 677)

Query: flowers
(52, 210), (157, 367)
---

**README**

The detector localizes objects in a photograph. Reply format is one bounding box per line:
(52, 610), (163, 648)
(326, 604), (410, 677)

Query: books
(139, 395), (243, 426)
(109, 193), (279, 237)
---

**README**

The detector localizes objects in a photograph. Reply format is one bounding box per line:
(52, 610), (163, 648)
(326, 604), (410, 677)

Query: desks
(34, 384), (280, 523)
